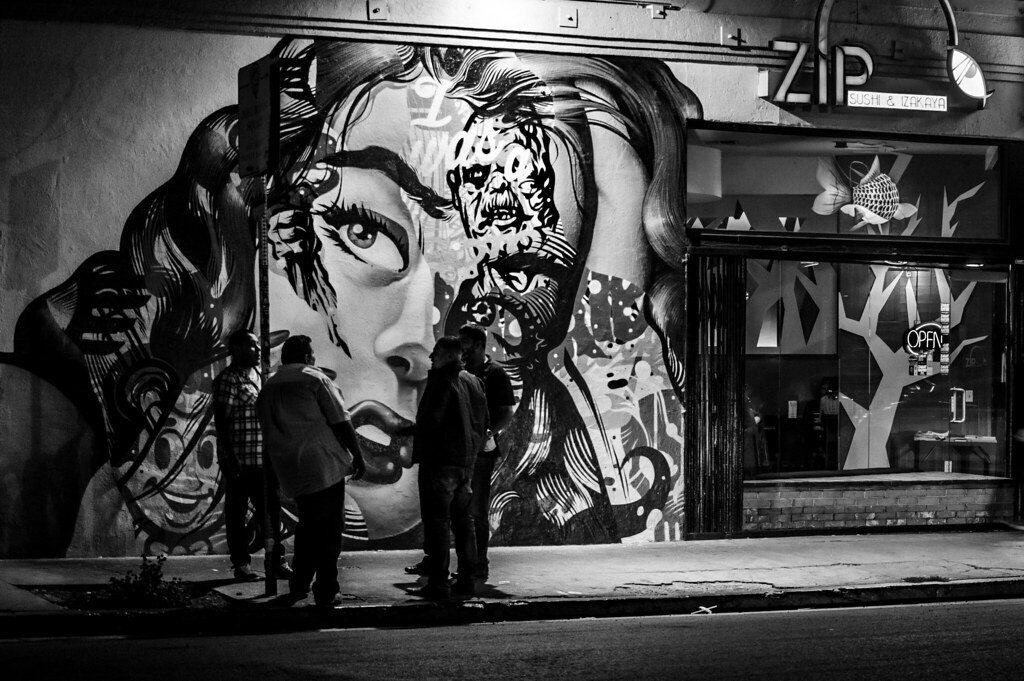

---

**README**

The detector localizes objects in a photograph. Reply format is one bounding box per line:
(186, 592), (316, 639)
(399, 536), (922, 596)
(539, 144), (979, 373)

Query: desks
(914, 432), (997, 475)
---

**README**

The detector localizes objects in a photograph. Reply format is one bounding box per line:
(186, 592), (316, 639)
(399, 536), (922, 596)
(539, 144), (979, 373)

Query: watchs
(486, 429), (492, 440)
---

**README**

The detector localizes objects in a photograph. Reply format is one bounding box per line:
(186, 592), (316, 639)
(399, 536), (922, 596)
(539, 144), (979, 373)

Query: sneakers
(265, 562), (293, 578)
(231, 561), (266, 581)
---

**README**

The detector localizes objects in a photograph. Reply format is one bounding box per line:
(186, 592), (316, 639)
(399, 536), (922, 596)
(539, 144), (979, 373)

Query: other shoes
(405, 556), (430, 574)
(406, 585), (451, 600)
(450, 583), (475, 600)
(451, 572), (488, 582)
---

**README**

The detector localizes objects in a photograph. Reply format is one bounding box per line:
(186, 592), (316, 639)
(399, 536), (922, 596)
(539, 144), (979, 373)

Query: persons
(406, 336), (491, 594)
(405, 327), (516, 580)
(212, 332), (293, 580)
(255, 335), (367, 605)
